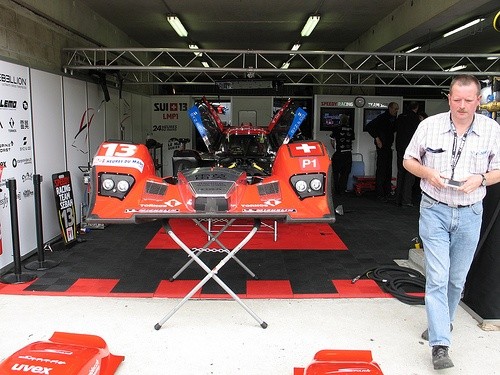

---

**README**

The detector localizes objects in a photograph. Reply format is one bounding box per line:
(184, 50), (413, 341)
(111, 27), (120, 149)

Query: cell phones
(444, 179), (465, 188)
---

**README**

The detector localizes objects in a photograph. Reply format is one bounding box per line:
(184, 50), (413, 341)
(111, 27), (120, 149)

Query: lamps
(280, 14), (322, 69)
(441, 15), (486, 38)
(403, 44), (422, 53)
(167, 15), (210, 68)
(444, 64), (468, 72)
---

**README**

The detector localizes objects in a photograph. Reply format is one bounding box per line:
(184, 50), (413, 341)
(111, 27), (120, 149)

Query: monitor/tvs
(320, 107), (355, 131)
(363, 108), (387, 132)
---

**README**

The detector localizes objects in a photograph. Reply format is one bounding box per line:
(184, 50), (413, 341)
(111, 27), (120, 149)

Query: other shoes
(336, 205), (343, 215)
(402, 203), (415, 208)
(377, 195), (389, 204)
(421, 322), (453, 341)
(432, 345), (454, 370)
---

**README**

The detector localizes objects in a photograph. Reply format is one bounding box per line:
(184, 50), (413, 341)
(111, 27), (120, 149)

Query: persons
(404, 76), (500, 370)
(367, 101), (429, 207)
(329, 114), (355, 216)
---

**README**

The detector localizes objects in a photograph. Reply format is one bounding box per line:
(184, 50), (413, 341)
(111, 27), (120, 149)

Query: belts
(423, 191), (474, 208)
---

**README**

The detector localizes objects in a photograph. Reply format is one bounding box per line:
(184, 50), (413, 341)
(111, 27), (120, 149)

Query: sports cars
(83, 96), (336, 224)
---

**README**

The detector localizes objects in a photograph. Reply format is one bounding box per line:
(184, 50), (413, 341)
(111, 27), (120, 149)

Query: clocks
(354, 97), (366, 108)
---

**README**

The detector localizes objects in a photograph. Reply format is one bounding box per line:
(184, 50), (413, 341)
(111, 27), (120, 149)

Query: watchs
(479, 174), (486, 188)
